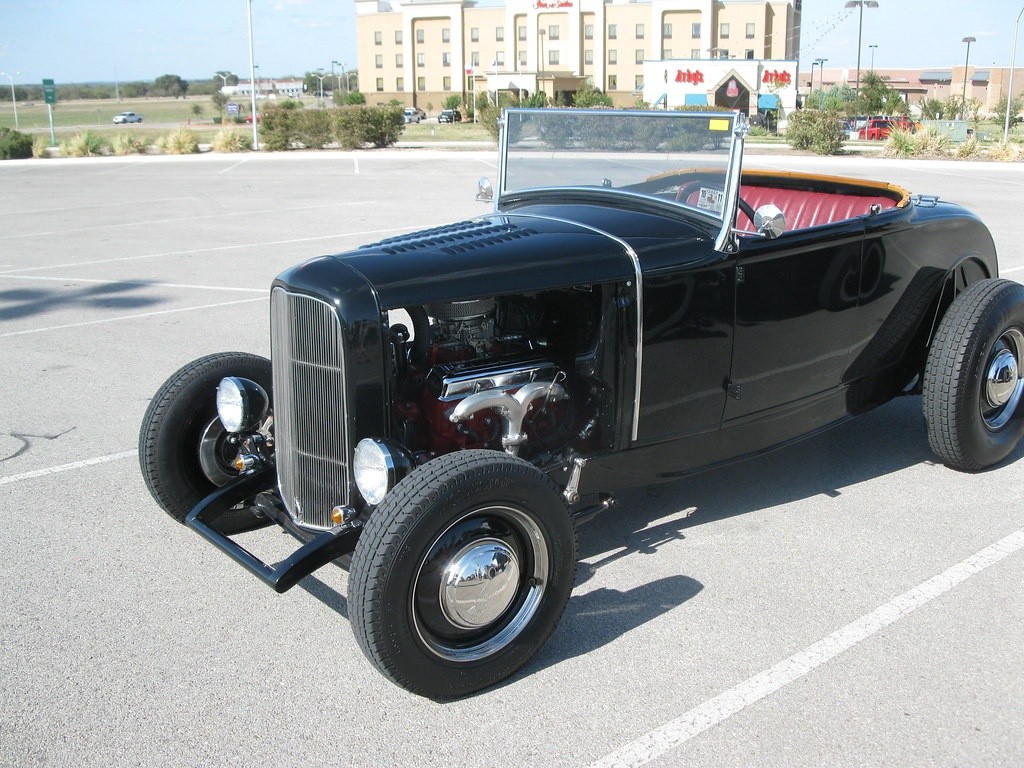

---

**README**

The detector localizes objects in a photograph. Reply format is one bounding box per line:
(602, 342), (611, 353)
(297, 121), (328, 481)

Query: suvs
(405, 107), (426, 118)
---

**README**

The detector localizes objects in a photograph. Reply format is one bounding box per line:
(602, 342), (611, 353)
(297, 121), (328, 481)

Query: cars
(245, 111), (271, 124)
(858, 119), (899, 141)
(113, 111), (142, 125)
(439, 111), (463, 125)
(847, 115), (917, 135)
(401, 111), (421, 123)
(839, 121), (852, 138)
(136, 110), (1023, 701)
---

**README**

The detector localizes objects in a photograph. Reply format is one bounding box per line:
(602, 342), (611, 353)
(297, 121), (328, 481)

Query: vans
(227, 102), (245, 115)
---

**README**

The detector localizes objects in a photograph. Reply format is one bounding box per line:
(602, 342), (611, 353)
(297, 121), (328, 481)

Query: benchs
(676, 181), (897, 234)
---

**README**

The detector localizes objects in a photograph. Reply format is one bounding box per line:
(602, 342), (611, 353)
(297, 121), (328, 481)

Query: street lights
(815, 58), (829, 91)
(844, 0), (880, 132)
(0, 70), (22, 131)
(214, 72), (237, 86)
(961, 36), (977, 121)
(868, 45), (878, 76)
(317, 61), (356, 94)
(311, 72), (333, 101)
(809, 62), (820, 96)
